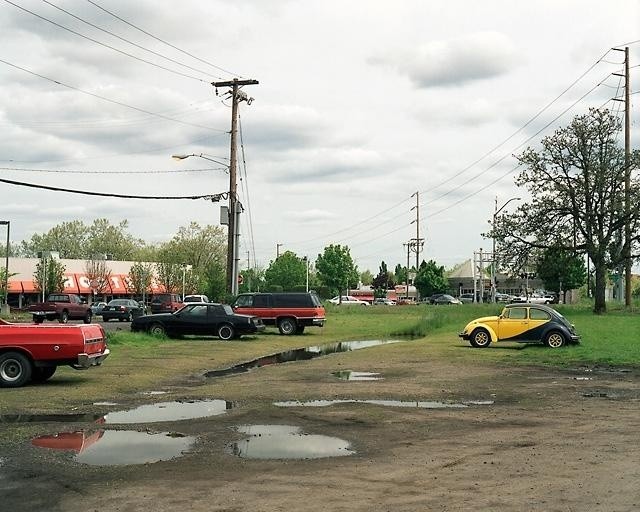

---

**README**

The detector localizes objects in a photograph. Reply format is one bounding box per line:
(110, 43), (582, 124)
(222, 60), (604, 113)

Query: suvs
(149, 292), (187, 314)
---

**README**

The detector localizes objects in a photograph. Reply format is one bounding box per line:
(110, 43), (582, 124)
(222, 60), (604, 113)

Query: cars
(129, 301), (265, 342)
(100, 298), (144, 323)
(328, 293), (371, 306)
(376, 290), (556, 306)
(136, 299), (149, 315)
(182, 294), (215, 310)
(0, 312), (112, 389)
(456, 302), (583, 348)
(90, 301), (108, 314)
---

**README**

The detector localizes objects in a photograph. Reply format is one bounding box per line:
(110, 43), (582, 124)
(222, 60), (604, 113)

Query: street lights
(491, 196), (523, 301)
(0, 218), (12, 317)
(170, 151), (238, 295)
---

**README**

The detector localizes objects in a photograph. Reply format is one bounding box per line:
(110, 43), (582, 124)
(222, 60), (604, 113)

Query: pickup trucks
(33, 416), (111, 456)
(27, 292), (95, 325)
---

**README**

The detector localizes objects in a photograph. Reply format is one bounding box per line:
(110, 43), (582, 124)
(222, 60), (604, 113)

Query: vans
(226, 289), (331, 334)
